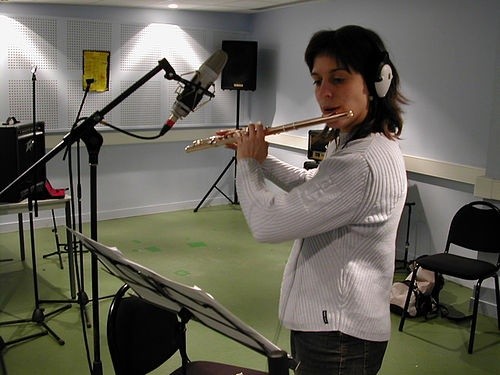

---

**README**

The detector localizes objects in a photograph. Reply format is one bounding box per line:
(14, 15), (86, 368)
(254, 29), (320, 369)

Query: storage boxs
(0, 116), (46, 203)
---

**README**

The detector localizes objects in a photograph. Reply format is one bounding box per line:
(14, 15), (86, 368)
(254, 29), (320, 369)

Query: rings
(242, 130), (249, 137)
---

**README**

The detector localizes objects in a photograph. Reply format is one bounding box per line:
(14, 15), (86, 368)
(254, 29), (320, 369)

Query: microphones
(159, 50), (228, 136)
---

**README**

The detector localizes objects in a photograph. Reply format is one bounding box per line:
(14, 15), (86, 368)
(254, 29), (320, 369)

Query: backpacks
(389, 263), (442, 318)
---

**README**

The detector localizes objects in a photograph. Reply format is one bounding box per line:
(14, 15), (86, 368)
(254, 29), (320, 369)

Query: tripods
(0, 65), (116, 346)
(193, 91), (241, 213)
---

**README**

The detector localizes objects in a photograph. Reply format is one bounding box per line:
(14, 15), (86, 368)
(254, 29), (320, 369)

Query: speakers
(221, 40), (258, 91)
(0, 120), (47, 203)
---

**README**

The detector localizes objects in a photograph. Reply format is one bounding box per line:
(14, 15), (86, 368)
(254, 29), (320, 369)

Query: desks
(0, 195), (75, 300)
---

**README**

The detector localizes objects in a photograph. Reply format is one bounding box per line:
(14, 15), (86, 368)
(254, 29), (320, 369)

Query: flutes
(185, 110), (354, 152)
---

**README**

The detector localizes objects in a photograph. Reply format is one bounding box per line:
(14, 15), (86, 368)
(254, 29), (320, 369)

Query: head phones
(367, 51), (393, 97)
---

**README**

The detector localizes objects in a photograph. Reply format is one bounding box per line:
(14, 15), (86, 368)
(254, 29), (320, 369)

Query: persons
(216, 25), (413, 375)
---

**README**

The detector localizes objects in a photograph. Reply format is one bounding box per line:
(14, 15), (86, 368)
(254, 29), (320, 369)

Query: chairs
(398, 199), (500, 354)
(107, 283), (269, 375)
